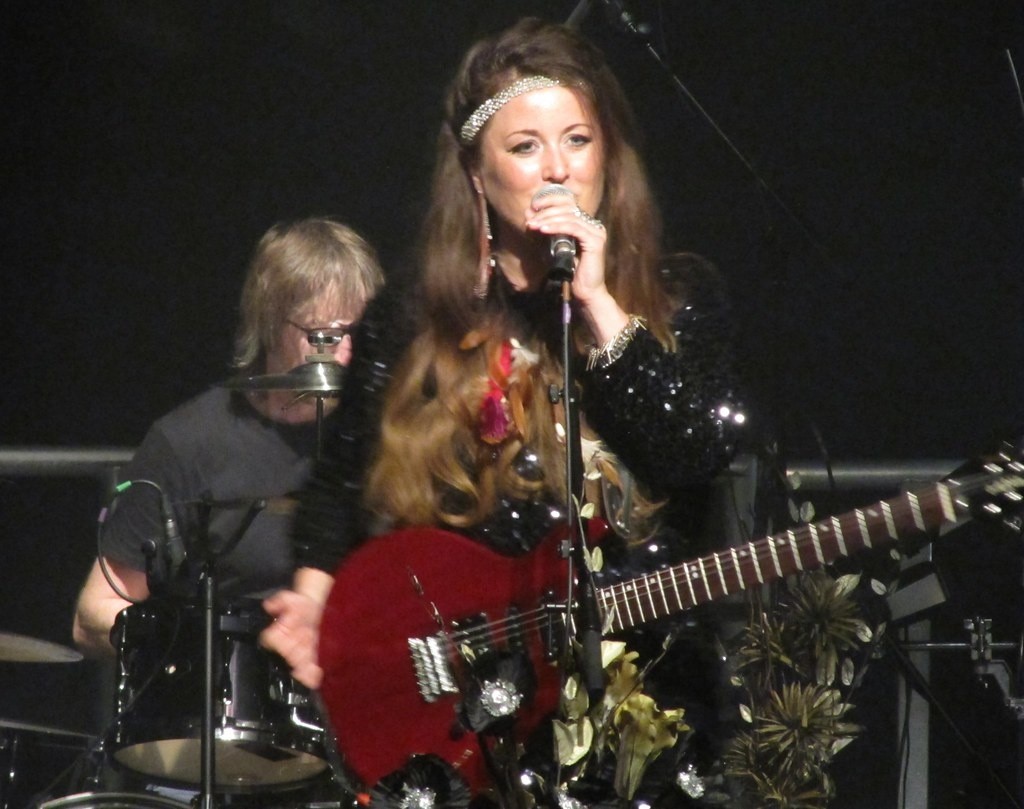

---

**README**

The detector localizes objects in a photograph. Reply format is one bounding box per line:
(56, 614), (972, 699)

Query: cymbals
(204, 361), (349, 396)
(0, 631), (85, 663)
(1, 718), (97, 740)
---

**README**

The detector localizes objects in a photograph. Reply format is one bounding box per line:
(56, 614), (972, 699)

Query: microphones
(533, 184), (577, 273)
(159, 496), (188, 581)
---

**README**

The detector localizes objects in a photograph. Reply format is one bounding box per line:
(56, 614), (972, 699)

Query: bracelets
(584, 314), (647, 371)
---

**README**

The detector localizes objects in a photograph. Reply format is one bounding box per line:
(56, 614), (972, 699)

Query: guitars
(315, 448), (1024, 800)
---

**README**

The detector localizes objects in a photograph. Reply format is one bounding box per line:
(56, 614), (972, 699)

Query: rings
(574, 206), (603, 229)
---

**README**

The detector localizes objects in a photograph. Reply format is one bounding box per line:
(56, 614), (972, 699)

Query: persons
(258, 14), (741, 809)
(67, 216), (388, 656)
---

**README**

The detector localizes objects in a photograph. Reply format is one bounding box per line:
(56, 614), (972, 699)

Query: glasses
(282, 315), (357, 348)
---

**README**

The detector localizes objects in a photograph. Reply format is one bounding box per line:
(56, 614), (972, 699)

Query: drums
(109, 592), (336, 795)
(41, 789), (192, 809)
